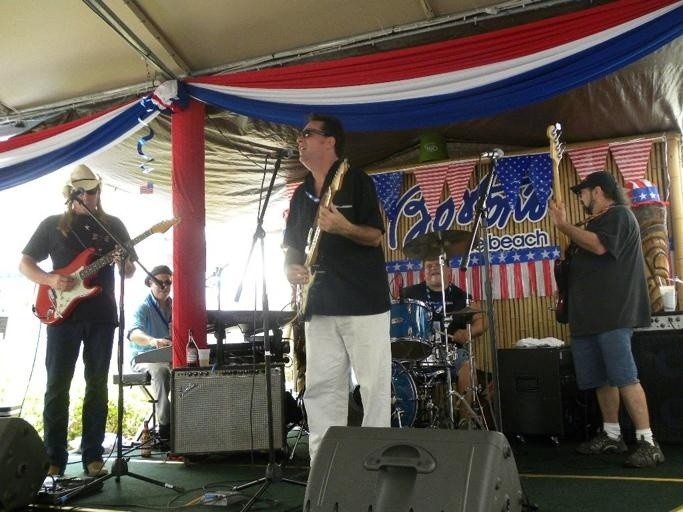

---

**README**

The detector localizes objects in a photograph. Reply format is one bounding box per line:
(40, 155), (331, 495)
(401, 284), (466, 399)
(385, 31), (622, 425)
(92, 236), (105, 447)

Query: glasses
(297, 127), (327, 138)
(73, 188), (96, 195)
(158, 279), (171, 285)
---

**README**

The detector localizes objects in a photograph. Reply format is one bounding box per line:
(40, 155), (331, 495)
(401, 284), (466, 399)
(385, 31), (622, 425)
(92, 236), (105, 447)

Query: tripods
(54, 253), (185, 504)
(232, 292), (306, 511)
(428, 256), (493, 428)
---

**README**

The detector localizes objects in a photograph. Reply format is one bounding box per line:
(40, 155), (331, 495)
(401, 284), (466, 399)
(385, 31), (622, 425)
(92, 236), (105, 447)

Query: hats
(569, 171), (614, 195)
(65, 164), (101, 193)
(144, 265), (172, 287)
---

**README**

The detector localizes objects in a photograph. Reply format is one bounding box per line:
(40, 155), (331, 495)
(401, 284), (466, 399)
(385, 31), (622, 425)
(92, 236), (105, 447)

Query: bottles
(184, 328), (197, 368)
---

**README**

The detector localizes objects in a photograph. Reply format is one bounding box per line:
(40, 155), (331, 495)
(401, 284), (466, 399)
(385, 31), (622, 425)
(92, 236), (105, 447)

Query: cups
(197, 349), (211, 367)
(660, 284), (676, 312)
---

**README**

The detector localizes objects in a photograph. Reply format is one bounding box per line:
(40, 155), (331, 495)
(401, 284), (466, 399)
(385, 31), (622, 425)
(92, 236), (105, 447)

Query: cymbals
(446, 308), (485, 314)
(403, 230), (478, 261)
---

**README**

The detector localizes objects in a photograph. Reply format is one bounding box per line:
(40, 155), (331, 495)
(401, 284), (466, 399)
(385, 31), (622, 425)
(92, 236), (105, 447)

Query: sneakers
(623, 435), (664, 469)
(575, 431), (628, 455)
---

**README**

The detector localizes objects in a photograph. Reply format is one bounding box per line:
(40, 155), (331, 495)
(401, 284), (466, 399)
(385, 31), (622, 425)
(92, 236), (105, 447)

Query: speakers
(172, 366), (286, 456)
(631, 330), (682, 442)
(499, 346), (564, 435)
(303, 426), (524, 512)
(0, 416), (50, 511)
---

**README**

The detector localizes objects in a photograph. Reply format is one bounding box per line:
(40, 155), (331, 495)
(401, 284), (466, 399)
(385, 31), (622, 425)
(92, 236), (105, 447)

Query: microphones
(270, 148), (300, 159)
(480, 148), (504, 159)
(64, 187), (84, 204)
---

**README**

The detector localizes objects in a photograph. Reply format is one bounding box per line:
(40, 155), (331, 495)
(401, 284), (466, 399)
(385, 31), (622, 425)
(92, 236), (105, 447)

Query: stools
(113, 372), (160, 442)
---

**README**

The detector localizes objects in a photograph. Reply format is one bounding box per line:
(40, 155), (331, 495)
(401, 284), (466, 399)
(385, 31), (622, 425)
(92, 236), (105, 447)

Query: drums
(390, 299), (433, 361)
(349, 359), (418, 428)
(414, 344), (457, 374)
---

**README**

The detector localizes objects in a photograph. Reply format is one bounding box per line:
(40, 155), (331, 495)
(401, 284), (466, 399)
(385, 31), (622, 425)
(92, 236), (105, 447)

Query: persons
(122, 264), (173, 446)
(546, 169), (666, 467)
(281, 110), (391, 472)
(396, 258), (491, 417)
(17, 163), (137, 478)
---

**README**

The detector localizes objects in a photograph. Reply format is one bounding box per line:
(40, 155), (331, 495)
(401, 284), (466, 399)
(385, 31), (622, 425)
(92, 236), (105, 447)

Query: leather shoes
(86, 461), (107, 477)
(47, 466), (59, 476)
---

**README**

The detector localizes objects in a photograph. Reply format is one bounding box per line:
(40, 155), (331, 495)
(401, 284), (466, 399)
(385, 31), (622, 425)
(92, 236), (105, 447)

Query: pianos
(134, 310), (296, 363)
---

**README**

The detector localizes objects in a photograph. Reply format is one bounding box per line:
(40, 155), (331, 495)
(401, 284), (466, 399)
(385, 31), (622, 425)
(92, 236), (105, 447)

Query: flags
(384, 241), (564, 306)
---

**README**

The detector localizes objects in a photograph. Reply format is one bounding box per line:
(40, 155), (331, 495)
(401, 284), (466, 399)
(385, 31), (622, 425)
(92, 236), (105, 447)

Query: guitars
(547, 122), (570, 324)
(295, 158), (350, 322)
(35, 218), (180, 325)
